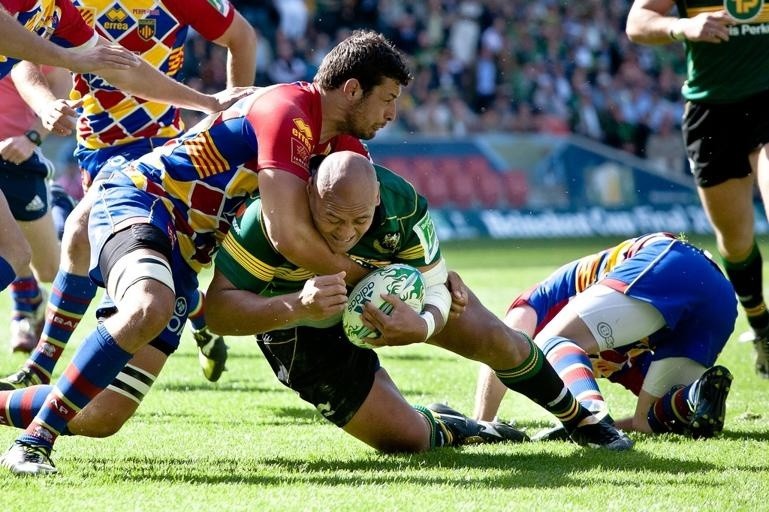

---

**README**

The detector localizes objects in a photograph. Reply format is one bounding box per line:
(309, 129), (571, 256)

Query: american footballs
(342, 263), (426, 349)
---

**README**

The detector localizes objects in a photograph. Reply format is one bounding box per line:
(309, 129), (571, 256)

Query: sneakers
(10, 287), (48, 352)
(531, 411), (614, 440)
(0, 371), (30, 390)
(429, 402), (529, 445)
(0, 440), (57, 474)
(752, 329), (769, 377)
(686, 366), (732, 440)
(565, 426), (627, 451)
(190, 291), (230, 382)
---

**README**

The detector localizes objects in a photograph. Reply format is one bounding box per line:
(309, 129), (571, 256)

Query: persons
(0, 0), (257, 393)
(203, 149), (631, 453)
(0, 0), (260, 291)
(0, 30), (413, 475)
(186, 0), (367, 91)
(624, 0), (769, 376)
(0, 60), (73, 356)
(367, 0), (688, 176)
(474, 230), (739, 441)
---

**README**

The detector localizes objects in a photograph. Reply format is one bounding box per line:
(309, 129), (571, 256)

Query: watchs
(23, 129), (43, 148)
